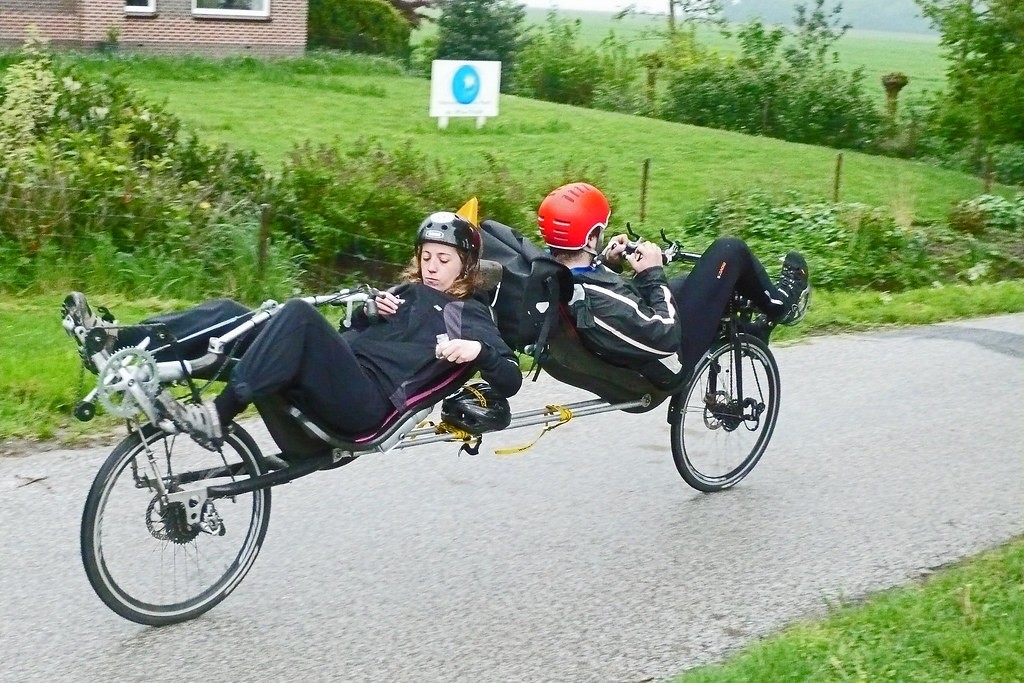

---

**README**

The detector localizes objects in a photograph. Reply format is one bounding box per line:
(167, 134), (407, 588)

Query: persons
(538, 183), (809, 391)
(62, 211), (523, 452)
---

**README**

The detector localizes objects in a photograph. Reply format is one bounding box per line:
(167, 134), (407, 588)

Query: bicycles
(59, 221), (781, 628)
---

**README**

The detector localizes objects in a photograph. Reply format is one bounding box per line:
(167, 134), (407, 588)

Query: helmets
(414, 212), (482, 274)
(538, 182), (611, 250)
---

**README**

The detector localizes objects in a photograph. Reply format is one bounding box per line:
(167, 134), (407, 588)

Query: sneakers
(780, 251), (809, 325)
(156, 394), (226, 452)
(62, 292), (119, 369)
(740, 313), (776, 360)
